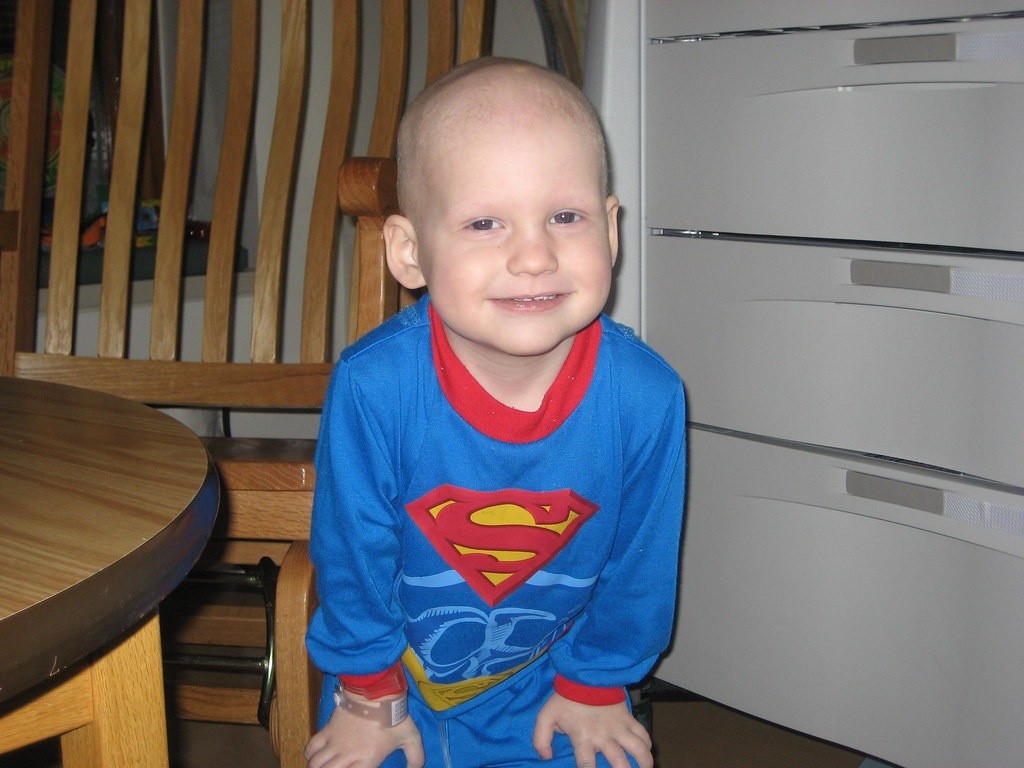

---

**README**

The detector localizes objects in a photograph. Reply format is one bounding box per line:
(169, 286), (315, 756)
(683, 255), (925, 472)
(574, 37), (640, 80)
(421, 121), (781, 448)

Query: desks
(0, 379), (228, 765)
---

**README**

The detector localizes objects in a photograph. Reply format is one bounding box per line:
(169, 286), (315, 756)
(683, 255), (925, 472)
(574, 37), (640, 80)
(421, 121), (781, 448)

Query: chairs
(0, 1), (501, 768)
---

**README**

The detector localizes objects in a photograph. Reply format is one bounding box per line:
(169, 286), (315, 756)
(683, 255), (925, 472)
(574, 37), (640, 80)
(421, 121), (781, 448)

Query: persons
(302, 56), (686, 768)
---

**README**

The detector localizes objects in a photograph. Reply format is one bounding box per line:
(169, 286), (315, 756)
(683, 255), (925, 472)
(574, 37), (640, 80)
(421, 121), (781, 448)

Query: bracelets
(334, 684), (408, 729)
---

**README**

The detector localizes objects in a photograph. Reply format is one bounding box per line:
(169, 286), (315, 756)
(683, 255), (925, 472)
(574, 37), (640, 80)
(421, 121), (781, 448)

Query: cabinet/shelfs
(606, 1), (1023, 767)
